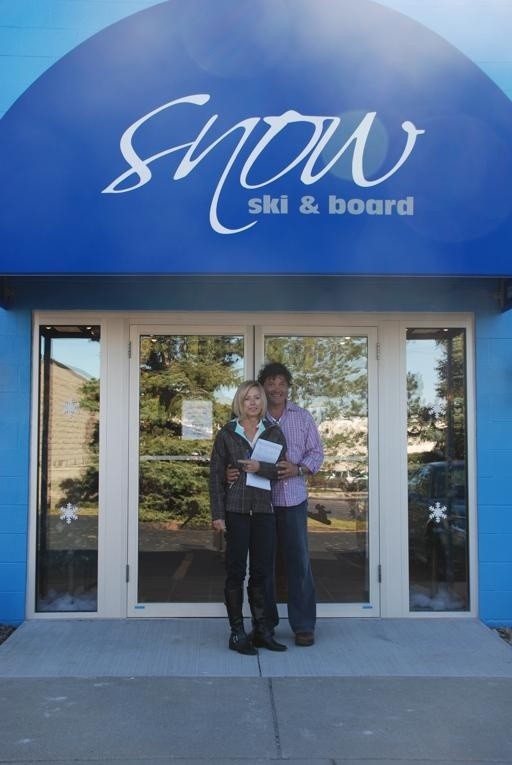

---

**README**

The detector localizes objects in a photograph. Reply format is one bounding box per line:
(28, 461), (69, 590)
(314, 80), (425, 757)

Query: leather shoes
(295, 632), (315, 646)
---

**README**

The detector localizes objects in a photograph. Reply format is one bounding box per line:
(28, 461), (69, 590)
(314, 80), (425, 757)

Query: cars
(408, 460), (467, 579)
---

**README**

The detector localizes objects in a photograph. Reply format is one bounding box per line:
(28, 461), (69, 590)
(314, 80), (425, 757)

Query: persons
(208, 364), (324, 655)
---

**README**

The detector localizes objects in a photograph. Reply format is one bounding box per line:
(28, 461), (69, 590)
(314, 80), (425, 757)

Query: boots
(224, 586), (288, 656)
